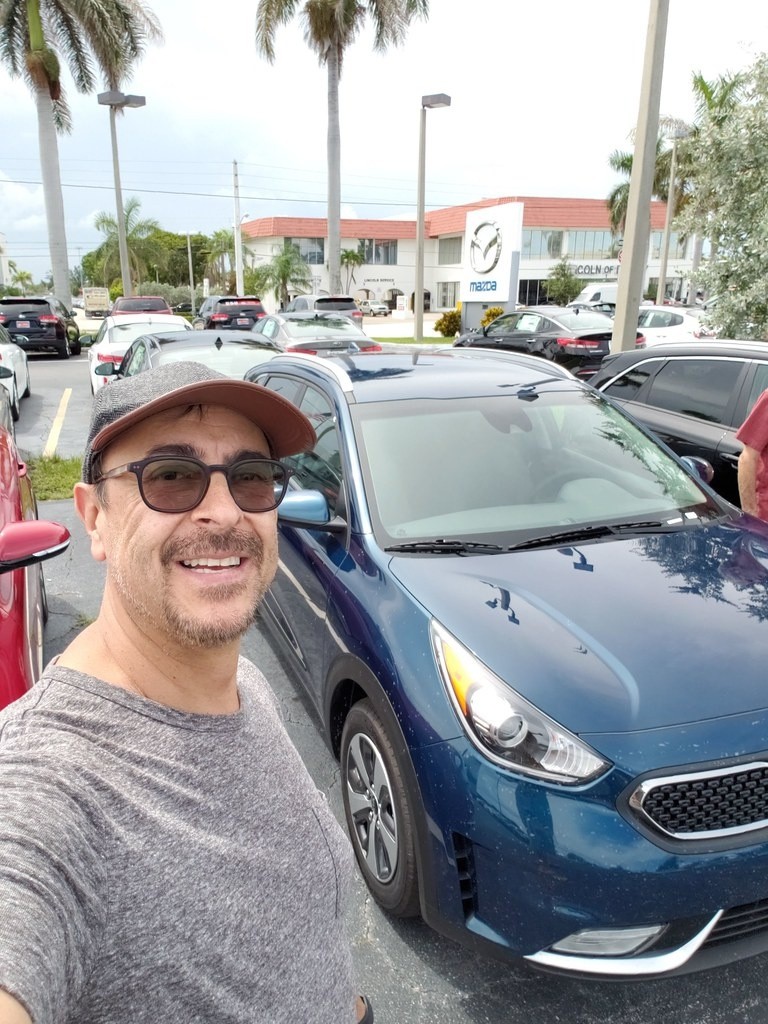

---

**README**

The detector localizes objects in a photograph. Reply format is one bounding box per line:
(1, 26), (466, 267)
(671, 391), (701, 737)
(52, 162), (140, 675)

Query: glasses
(92, 456), (296, 513)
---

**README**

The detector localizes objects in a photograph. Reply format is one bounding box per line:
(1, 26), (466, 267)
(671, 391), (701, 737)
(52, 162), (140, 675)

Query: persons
(2, 356), (379, 1023)
(734, 386), (768, 525)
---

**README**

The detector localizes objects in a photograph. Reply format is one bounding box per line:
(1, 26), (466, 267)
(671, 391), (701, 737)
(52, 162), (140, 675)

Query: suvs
(0, 294), (81, 359)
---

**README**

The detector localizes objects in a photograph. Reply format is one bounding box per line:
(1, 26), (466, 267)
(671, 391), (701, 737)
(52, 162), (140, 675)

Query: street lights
(414, 93), (451, 340)
(656, 123), (703, 305)
(97, 91), (145, 296)
(234, 212), (250, 295)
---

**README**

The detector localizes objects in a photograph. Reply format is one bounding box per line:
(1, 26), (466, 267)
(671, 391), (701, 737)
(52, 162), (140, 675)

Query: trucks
(566, 283), (620, 307)
(83, 286), (110, 318)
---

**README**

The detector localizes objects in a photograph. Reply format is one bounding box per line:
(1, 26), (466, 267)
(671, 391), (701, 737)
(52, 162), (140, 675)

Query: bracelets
(357, 993), (374, 1024)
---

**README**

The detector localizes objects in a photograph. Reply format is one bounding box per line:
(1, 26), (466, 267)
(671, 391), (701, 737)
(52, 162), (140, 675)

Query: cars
(110, 294), (173, 317)
(194, 295), (269, 332)
(611, 304), (730, 350)
(643, 295), (721, 308)
(71, 291), (115, 312)
(578, 338), (768, 510)
(278, 292), (363, 331)
(244, 347), (768, 982)
(93, 329), (285, 385)
(569, 302), (617, 317)
(251, 310), (383, 357)
(357, 299), (389, 317)
(1, 321), (31, 425)
(0, 363), (17, 445)
(0, 423), (71, 712)
(453, 305), (648, 382)
(77, 313), (197, 397)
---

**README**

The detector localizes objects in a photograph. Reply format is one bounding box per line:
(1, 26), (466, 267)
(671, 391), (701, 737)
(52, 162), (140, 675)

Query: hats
(82, 361), (318, 482)
